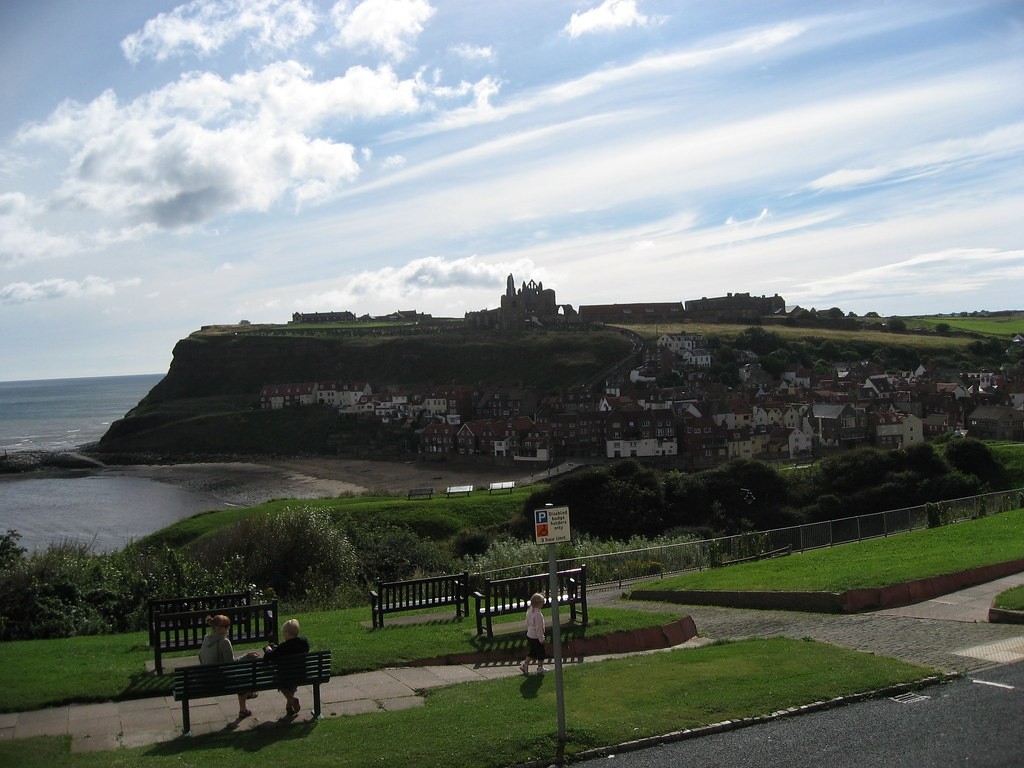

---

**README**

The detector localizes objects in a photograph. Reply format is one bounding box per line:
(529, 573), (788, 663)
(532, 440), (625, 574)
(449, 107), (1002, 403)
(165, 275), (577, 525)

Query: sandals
(247, 692), (258, 699)
(238, 710), (251, 718)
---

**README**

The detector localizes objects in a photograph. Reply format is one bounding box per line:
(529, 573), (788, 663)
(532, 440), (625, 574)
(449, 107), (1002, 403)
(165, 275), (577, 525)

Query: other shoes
(286, 704), (293, 714)
(293, 698), (300, 712)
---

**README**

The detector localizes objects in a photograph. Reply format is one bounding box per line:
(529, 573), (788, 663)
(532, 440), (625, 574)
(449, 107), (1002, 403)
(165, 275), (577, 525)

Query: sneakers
(519, 664), (528, 674)
(537, 667), (549, 673)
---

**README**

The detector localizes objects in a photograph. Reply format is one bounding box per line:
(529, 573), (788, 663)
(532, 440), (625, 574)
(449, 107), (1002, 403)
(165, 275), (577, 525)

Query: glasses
(220, 624), (229, 628)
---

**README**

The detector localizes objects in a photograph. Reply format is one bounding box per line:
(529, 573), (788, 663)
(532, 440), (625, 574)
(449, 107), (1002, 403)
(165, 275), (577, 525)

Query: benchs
(488, 481), (516, 495)
(148, 590), (280, 674)
(171, 650), (332, 733)
(370, 570), (470, 628)
(473, 564), (588, 641)
(444, 485), (474, 498)
(407, 487), (434, 500)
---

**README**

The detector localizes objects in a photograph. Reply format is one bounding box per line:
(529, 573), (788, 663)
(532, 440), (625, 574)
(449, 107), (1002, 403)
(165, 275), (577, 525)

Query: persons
(197, 615), (260, 718)
(519, 594), (550, 674)
(264, 619), (310, 716)
